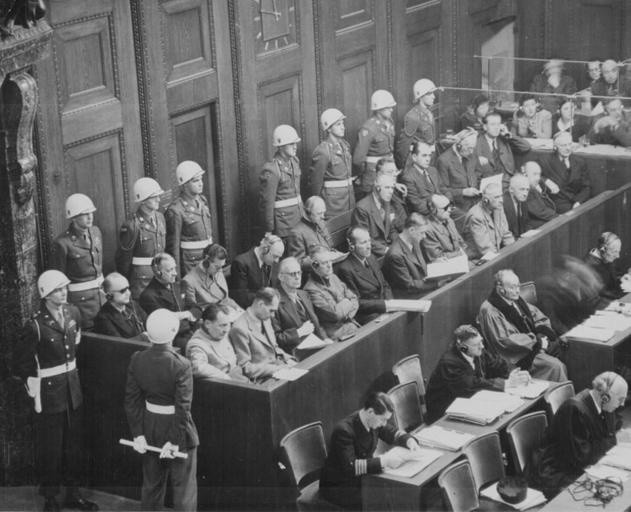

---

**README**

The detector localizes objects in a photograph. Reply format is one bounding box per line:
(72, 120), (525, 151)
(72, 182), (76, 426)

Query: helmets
(144, 306), (182, 345)
(370, 89), (397, 112)
(37, 270), (71, 300)
(412, 78), (440, 102)
(176, 160), (206, 187)
(64, 193), (97, 220)
(272, 124), (302, 148)
(320, 108), (347, 132)
(133, 177), (167, 205)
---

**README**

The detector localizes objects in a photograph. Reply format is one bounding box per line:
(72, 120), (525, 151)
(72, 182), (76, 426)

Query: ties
(363, 259), (376, 277)
(120, 307), (136, 327)
(338, 137), (347, 154)
(260, 319), (267, 339)
(422, 168), (435, 188)
(608, 82), (613, 93)
(193, 195), (204, 215)
(490, 210), (496, 224)
(412, 244), (420, 262)
(459, 156), (469, 173)
(293, 294), (311, 319)
(148, 215), (159, 232)
(443, 220), (451, 235)
(384, 120), (393, 135)
(423, 108), (434, 127)
(287, 155), (298, 181)
(382, 200), (393, 237)
(600, 411), (610, 440)
(490, 137), (500, 157)
(316, 228), (328, 248)
(509, 301), (516, 308)
(325, 277), (332, 291)
(55, 310), (67, 331)
(208, 274), (226, 298)
(81, 228), (91, 248)
(262, 263), (270, 286)
(473, 355), (483, 376)
(517, 202), (525, 233)
(368, 428), (373, 443)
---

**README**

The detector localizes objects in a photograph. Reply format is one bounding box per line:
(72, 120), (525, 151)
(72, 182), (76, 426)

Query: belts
(409, 144), (436, 155)
(323, 177), (355, 188)
(366, 154), (395, 164)
(37, 357), (78, 379)
(68, 273), (105, 293)
(274, 194), (302, 211)
(180, 237), (214, 251)
(130, 256), (155, 266)
(144, 399), (177, 415)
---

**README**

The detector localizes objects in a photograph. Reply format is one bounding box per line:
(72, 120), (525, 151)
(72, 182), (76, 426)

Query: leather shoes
(43, 496), (60, 512)
(63, 495), (100, 512)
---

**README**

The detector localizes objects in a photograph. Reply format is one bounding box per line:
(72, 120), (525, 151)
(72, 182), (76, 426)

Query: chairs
(520, 280), (539, 306)
(461, 430), (547, 511)
(280, 420), (342, 511)
(386, 380), (425, 435)
(438, 459), (480, 512)
(392, 353), (428, 415)
(543, 378), (574, 415)
(503, 410), (549, 477)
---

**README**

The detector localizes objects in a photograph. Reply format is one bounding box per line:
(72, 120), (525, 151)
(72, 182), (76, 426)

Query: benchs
(379, 215), (467, 268)
(221, 209), (355, 278)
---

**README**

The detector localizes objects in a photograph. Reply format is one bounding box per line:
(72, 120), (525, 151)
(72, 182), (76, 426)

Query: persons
(583, 231), (630, 298)
(351, 173), (398, 256)
(436, 128), (482, 211)
(256, 124), (303, 242)
(138, 252), (203, 323)
(230, 285), (298, 384)
(270, 256), (334, 359)
(122, 308), (197, 512)
(320, 391), (420, 512)
(336, 225), (394, 326)
(400, 141), (467, 233)
(460, 94), (489, 135)
(11, 270), (99, 512)
(478, 269), (568, 383)
(588, 96), (631, 146)
(94, 271), (149, 339)
(552, 98), (584, 143)
(527, 369), (628, 501)
(538, 130), (592, 214)
(165, 160), (213, 283)
(529, 57), (576, 106)
(477, 111), (531, 190)
(591, 59), (631, 108)
(182, 243), (229, 311)
(501, 173), (530, 238)
(353, 89), (396, 197)
(287, 195), (334, 270)
(229, 234), (285, 309)
(302, 246), (363, 339)
(461, 183), (515, 257)
(425, 329), (531, 423)
(522, 160), (562, 228)
(573, 59), (601, 108)
(115, 177), (167, 301)
(511, 93), (552, 140)
(382, 211), (452, 300)
(420, 193), (479, 279)
(307, 107), (355, 221)
(54, 192), (105, 334)
(376, 156), (409, 232)
(185, 302), (250, 386)
(396, 78), (438, 168)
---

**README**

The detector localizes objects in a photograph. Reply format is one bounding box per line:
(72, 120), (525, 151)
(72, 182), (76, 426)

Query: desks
(566, 292), (631, 392)
(437, 135), (631, 196)
(362, 378), (572, 512)
(535, 440), (630, 511)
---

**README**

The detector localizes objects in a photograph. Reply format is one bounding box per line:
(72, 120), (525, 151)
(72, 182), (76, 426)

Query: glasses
(280, 269), (305, 280)
(110, 286), (132, 294)
(436, 201), (455, 212)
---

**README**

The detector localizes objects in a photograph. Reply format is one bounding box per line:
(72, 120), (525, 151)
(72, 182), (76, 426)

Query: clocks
(253, 0), (296, 51)
